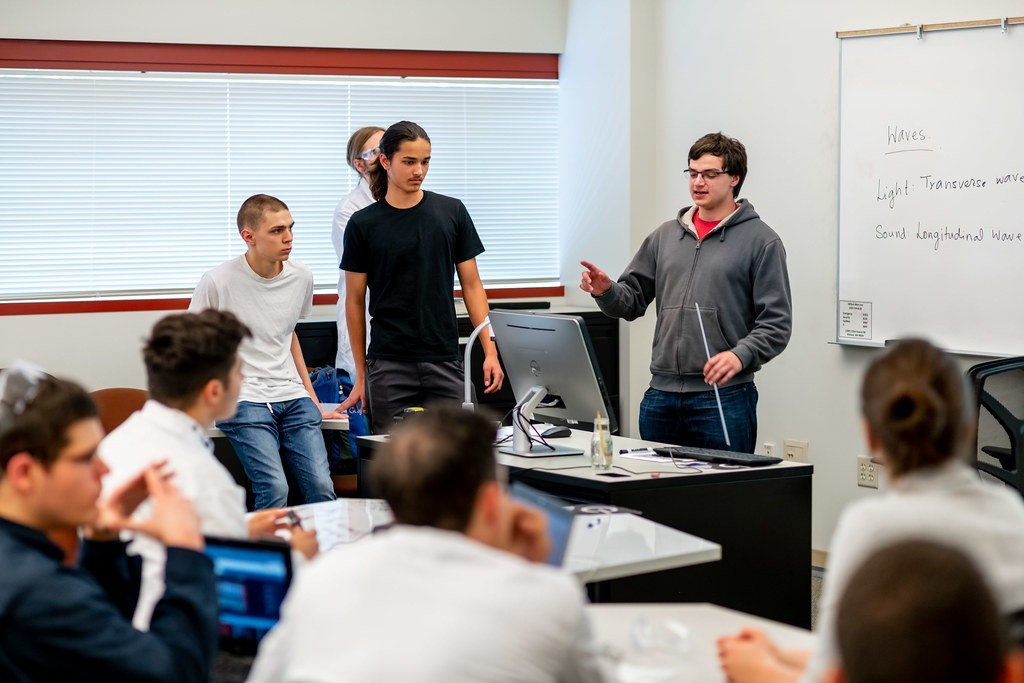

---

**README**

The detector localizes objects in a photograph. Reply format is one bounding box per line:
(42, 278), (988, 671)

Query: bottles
(591, 418), (613, 470)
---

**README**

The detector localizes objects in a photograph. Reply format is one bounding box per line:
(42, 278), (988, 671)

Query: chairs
(961, 354), (1024, 500)
(86, 378), (153, 439)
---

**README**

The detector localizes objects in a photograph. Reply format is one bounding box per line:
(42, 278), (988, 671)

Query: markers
(619, 446), (653, 455)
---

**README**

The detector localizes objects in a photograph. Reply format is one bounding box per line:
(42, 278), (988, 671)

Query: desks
(201, 398), (353, 439)
(230, 494), (717, 592)
(587, 600), (835, 681)
(355, 407), (812, 629)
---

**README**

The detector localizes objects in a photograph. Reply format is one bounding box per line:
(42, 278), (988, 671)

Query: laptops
(504, 482), (574, 568)
(198, 535), (284, 682)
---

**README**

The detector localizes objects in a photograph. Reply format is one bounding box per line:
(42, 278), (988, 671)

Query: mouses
(541, 426), (571, 438)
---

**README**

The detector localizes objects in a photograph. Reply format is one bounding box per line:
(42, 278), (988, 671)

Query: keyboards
(653, 446), (783, 467)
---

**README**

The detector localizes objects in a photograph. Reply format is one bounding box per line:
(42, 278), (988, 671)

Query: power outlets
(856, 455), (878, 487)
(784, 440), (809, 462)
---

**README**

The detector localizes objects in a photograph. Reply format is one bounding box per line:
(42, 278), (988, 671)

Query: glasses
(681, 168), (730, 179)
(0, 358), (64, 413)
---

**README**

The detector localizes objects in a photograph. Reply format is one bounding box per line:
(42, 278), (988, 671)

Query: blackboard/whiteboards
(828, 15), (1023, 360)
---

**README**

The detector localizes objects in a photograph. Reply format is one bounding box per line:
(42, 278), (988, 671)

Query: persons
(579, 132), (792, 454)
(189, 195), (336, 511)
(718, 336), (1024, 683)
(247, 406), (613, 683)
(0, 365), (218, 683)
(334, 122), (504, 434)
(99, 307), (318, 641)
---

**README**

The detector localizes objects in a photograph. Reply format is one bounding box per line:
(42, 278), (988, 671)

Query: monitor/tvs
(489, 309), (619, 457)
